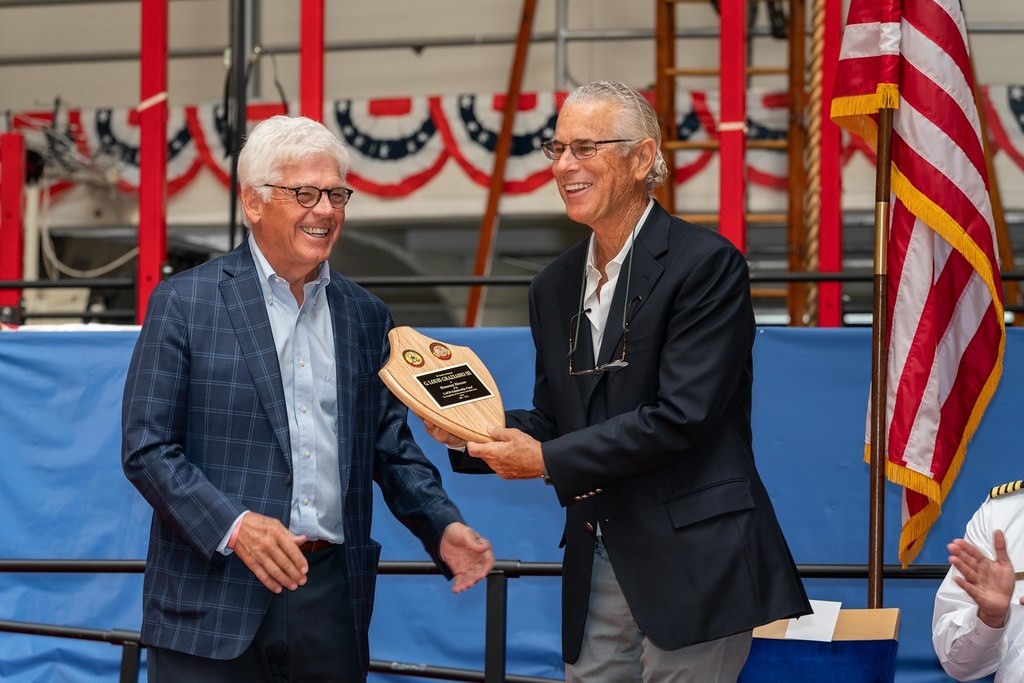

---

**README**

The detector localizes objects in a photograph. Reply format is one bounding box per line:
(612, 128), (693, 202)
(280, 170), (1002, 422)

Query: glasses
(262, 183), (354, 210)
(541, 138), (634, 162)
(568, 294), (643, 377)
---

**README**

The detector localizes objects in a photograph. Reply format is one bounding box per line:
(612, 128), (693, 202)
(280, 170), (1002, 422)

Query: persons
(928, 481), (1024, 683)
(416, 79), (813, 683)
(119, 115), (494, 683)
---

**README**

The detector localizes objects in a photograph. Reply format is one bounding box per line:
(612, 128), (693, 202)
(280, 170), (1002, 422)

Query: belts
(299, 539), (335, 553)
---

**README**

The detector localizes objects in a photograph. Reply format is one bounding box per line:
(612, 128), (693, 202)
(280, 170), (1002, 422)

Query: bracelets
(228, 518), (243, 549)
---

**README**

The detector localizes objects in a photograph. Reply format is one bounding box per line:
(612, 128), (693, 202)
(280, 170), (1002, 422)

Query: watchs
(540, 467), (552, 485)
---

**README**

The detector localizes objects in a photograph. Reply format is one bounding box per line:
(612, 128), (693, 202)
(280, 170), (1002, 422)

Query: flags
(829, 0), (1008, 572)
